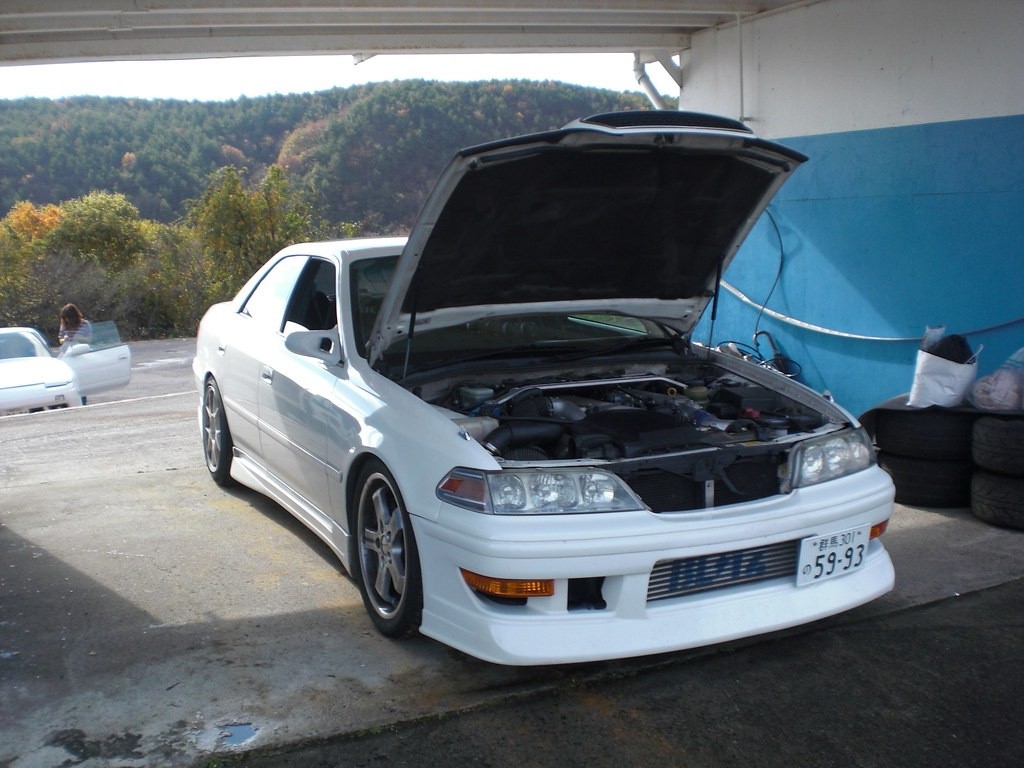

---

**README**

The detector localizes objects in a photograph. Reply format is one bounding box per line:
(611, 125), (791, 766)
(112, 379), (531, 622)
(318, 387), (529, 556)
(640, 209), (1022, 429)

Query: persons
(58, 304), (92, 405)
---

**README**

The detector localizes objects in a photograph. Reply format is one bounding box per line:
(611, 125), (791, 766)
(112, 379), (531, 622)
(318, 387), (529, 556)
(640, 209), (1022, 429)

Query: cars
(0, 320), (131, 415)
(193, 109), (896, 666)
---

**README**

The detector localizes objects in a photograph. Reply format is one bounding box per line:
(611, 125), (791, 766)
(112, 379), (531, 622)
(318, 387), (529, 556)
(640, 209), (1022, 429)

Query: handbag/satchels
(965, 347), (1024, 415)
(905, 344), (984, 408)
